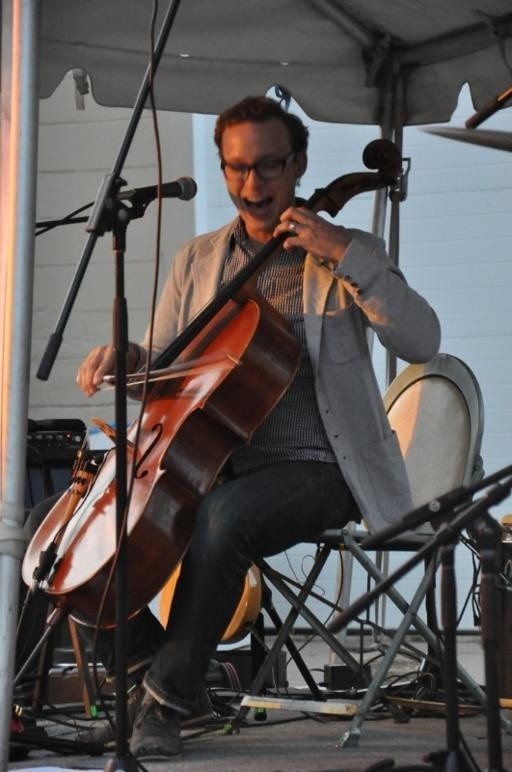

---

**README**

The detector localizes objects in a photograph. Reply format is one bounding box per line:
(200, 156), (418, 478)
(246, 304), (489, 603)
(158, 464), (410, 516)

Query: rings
(289, 222), (296, 233)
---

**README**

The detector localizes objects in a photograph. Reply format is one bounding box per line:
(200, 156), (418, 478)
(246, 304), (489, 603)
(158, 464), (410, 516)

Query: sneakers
(76, 680), (144, 755)
(128, 691), (185, 762)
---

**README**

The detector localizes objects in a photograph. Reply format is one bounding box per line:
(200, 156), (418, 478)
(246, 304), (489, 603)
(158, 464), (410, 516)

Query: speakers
(12, 415), (103, 668)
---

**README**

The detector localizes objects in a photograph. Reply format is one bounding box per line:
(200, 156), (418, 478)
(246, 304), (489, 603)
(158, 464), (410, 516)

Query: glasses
(220, 149), (297, 182)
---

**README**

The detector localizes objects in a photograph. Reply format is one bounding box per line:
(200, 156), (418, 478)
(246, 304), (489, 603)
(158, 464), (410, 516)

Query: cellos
(22, 139), (404, 629)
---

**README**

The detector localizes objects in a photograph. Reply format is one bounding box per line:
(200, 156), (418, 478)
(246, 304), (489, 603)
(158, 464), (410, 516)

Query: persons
(74, 95), (440, 760)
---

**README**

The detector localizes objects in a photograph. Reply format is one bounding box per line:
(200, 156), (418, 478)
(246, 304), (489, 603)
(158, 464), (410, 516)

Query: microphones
(117, 175), (198, 201)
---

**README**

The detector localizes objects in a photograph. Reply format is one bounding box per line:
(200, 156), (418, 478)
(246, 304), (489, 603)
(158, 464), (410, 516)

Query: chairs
(222, 351), (512, 746)
